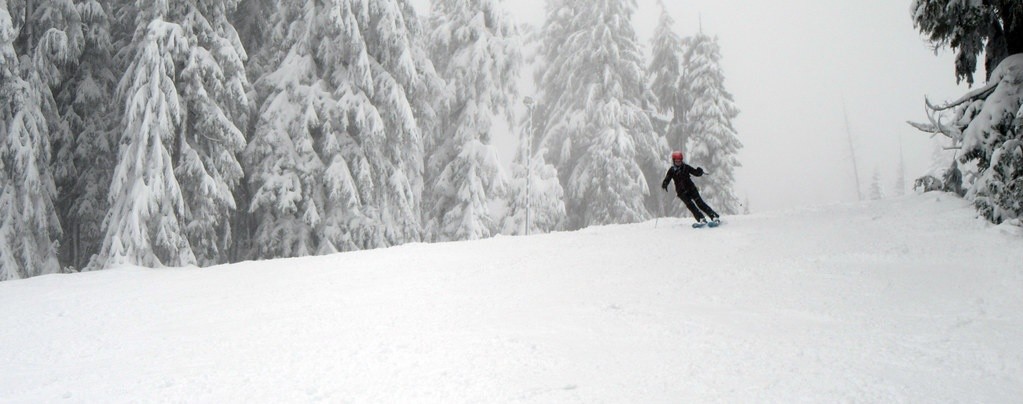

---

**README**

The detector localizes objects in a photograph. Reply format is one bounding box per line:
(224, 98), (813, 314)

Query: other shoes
(713, 215), (718, 221)
(699, 217), (706, 222)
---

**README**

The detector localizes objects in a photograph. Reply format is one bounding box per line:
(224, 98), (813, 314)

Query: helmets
(671, 152), (683, 159)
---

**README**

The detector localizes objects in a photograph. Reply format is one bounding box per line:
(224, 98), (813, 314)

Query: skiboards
(692, 219), (722, 228)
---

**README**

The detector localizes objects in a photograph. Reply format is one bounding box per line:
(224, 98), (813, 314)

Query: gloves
(662, 183), (667, 189)
(697, 167), (703, 174)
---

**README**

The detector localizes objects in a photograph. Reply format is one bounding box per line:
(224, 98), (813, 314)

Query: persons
(661, 151), (720, 223)
